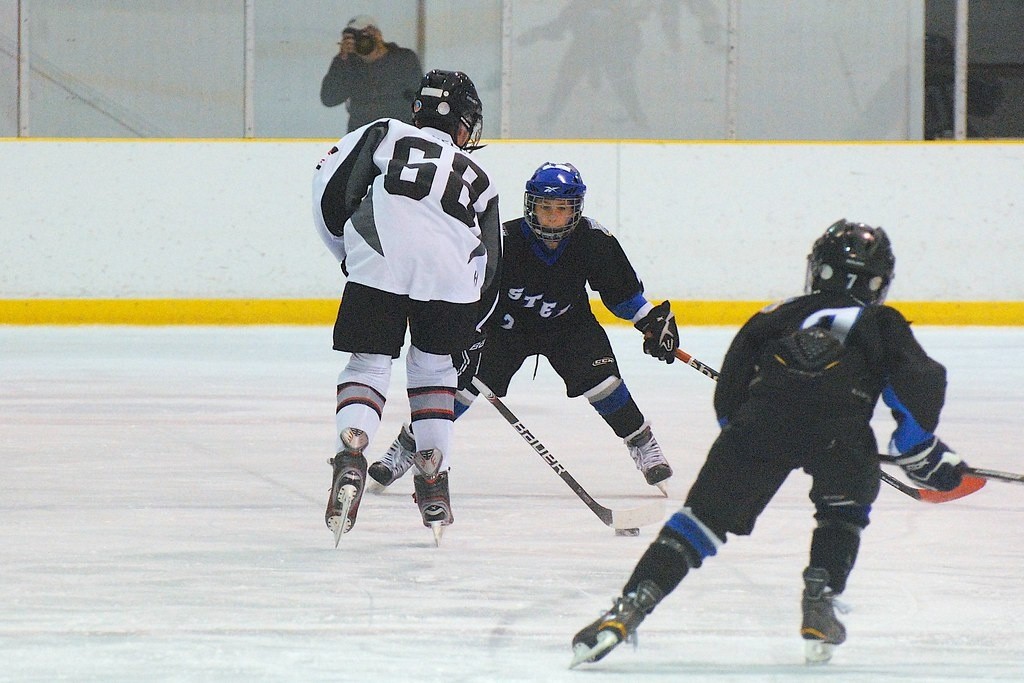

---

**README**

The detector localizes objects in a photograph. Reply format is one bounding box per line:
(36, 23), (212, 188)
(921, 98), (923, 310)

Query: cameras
(342, 28), (375, 55)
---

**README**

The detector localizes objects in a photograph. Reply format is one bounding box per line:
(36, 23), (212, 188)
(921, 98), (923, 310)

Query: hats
(348, 14), (380, 30)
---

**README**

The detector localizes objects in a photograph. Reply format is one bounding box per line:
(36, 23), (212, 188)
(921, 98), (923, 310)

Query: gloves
(340, 256), (349, 278)
(451, 331), (487, 391)
(634, 300), (679, 364)
(897, 435), (971, 492)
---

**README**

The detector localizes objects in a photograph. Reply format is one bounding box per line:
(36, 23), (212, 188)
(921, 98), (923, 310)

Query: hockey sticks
(647, 332), (987, 504)
(465, 372), (667, 529)
(879, 454), (1024, 487)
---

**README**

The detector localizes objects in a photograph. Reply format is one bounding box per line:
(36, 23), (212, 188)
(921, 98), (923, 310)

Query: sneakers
(364, 423), (417, 495)
(325, 427), (369, 548)
(412, 448), (454, 548)
(800, 567), (846, 665)
(568, 579), (664, 670)
(623, 421), (673, 498)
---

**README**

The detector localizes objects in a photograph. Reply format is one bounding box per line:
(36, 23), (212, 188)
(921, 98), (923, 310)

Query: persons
(313, 69), (504, 548)
(320, 16), (424, 135)
(569, 220), (969, 673)
(365, 163), (679, 502)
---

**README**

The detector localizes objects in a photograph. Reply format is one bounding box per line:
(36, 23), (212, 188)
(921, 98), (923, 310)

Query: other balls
(615, 527), (643, 537)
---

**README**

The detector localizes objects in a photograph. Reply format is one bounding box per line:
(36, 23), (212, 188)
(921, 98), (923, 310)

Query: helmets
(413, 69), (483, 118)
(812, 218), (896, 279)
(526, 161), (586, 240)
